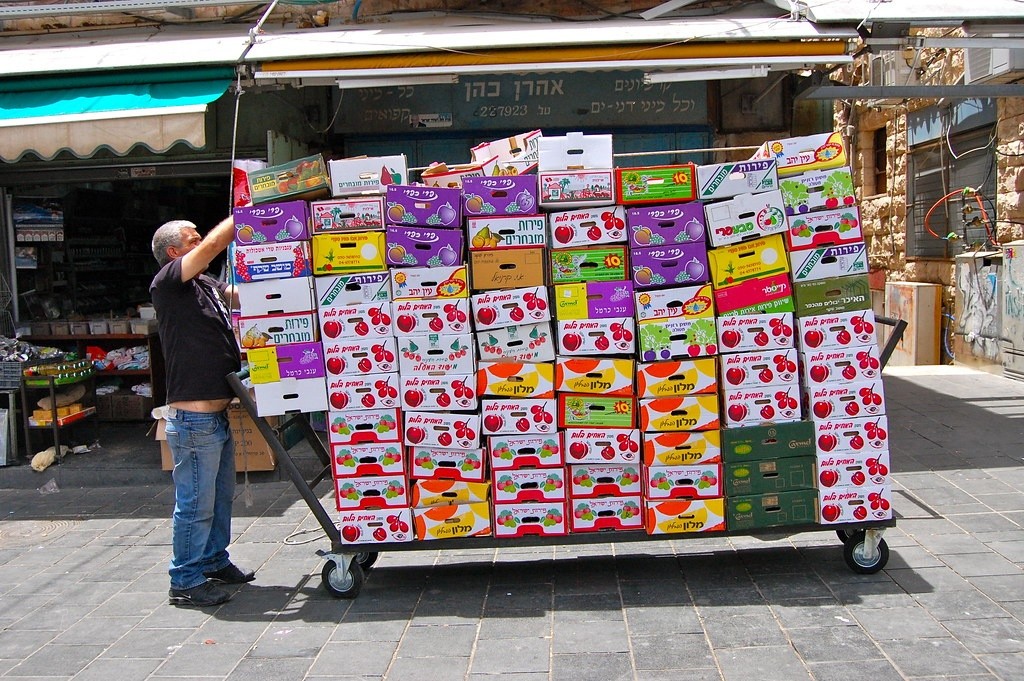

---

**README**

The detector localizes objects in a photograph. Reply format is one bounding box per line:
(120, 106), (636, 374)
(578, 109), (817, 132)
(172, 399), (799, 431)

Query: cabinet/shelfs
(17, 334), (160, 423)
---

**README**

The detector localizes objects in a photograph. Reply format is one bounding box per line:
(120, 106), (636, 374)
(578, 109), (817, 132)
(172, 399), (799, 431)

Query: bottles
(23, 359), (93, 379)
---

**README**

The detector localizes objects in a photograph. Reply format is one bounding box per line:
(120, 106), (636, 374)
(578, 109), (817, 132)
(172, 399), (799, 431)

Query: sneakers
(202, 561), (255, 583)
(168, 579), (231, 606)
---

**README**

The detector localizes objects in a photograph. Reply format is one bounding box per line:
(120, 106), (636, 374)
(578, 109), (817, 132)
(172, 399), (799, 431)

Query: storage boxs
(0, 306), (283, 472)
(232, 115), (894, 543)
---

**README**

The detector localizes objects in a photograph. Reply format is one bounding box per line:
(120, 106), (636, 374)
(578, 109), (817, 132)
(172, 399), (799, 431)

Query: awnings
(0, 68), (237, 163)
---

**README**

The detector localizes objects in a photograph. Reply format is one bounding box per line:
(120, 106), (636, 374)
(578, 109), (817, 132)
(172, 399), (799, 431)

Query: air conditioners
(965, 28), (1024, 90)
(868, 46), (922, 106)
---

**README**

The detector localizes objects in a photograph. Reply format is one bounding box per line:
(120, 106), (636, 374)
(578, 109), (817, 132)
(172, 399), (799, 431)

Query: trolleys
(223, 314), (910, 601)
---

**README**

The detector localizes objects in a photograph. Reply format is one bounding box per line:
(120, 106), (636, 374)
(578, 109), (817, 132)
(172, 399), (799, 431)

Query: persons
(149, 215), (255, 609)
